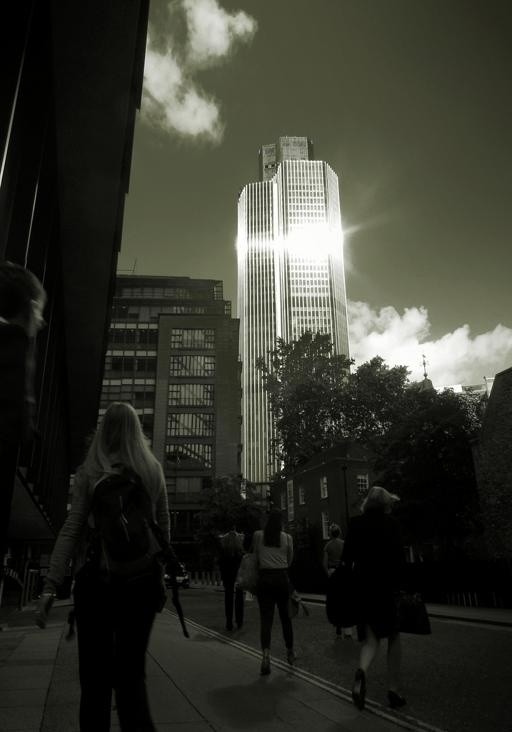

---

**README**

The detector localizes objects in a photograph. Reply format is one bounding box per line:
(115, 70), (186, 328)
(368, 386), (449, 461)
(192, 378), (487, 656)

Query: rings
(35, 611), (41, 617)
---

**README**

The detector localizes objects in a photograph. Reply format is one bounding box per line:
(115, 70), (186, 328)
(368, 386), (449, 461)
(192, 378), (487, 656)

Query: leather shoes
(288, 649), (300, 665)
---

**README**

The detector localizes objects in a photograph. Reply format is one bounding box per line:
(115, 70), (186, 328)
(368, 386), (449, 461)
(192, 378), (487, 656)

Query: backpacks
(86, 462), (166, 599)
(242, 552), (257, 577)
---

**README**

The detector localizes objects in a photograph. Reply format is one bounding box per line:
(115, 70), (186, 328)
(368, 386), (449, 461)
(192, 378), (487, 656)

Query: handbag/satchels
(399, 591), (432, 635)
(288, 589), (310, 618)
(326, 558), (357, 629)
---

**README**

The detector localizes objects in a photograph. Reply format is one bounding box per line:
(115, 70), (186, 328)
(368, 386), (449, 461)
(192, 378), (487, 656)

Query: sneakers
(225, 621), (244, 631)
(333, 631), (353, 643)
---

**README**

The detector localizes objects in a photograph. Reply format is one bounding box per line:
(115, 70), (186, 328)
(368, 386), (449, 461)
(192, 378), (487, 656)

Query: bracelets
(38, 593), (57, 599)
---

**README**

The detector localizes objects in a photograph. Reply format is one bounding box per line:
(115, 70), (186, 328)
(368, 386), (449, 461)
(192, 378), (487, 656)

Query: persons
(251, 509), (296, 675)
(323, 523), (354, 643)
(1, 260), (51, 589)
(36, 402), (177, 732)
(338, 486), (432, 710)
(213, 530), (246, 632)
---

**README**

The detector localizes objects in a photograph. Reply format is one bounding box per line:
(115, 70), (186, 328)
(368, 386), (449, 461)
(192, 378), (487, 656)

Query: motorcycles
(163, 562), (189, 589)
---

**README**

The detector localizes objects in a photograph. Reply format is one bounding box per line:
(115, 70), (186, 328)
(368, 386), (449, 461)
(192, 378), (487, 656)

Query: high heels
(352, 668), (366, 711)
(259, 649), (270, 678)
(387, 690), (408, 709)
